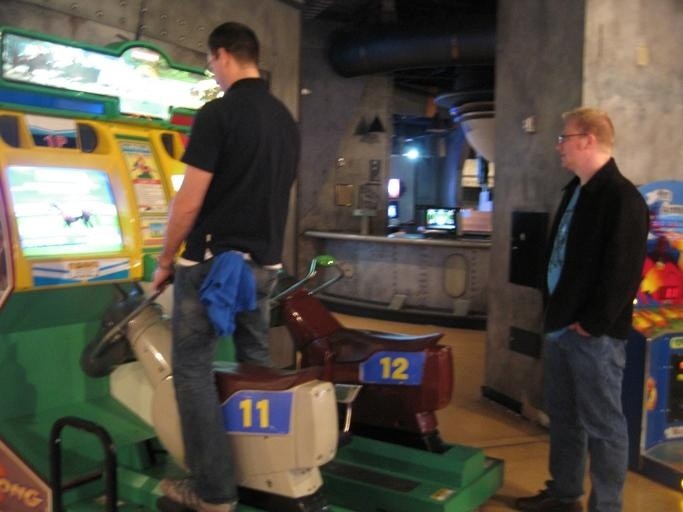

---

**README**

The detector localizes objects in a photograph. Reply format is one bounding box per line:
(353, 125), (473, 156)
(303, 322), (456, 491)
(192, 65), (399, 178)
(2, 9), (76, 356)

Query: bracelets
(156, 257), (174, 270)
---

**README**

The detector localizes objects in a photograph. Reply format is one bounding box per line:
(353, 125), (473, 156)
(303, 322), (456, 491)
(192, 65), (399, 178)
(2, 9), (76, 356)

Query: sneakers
(517, 488), (582, 512)
(160, 480), (238, 512)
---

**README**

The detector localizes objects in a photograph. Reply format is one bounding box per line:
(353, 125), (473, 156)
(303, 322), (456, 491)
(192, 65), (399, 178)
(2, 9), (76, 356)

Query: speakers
(507, 210), (555, 290)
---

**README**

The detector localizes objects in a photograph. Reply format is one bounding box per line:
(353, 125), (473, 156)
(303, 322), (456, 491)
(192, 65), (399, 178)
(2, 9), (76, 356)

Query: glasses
(558, 134), (587, 142)
(204, 56), (219, 77)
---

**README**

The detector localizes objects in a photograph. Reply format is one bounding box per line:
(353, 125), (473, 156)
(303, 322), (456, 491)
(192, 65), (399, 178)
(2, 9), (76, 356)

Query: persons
(150, 22), (303, 511)
(514, 108), (650, 512)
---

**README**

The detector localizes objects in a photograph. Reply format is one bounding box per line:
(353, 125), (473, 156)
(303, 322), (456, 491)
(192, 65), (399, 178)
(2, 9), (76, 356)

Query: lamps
(351, 113), (386, 136)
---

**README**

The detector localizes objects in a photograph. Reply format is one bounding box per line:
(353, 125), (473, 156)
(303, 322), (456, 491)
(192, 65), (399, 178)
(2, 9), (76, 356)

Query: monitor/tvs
(422, 205), (459, 232)
(5, 164), (124, 258)
